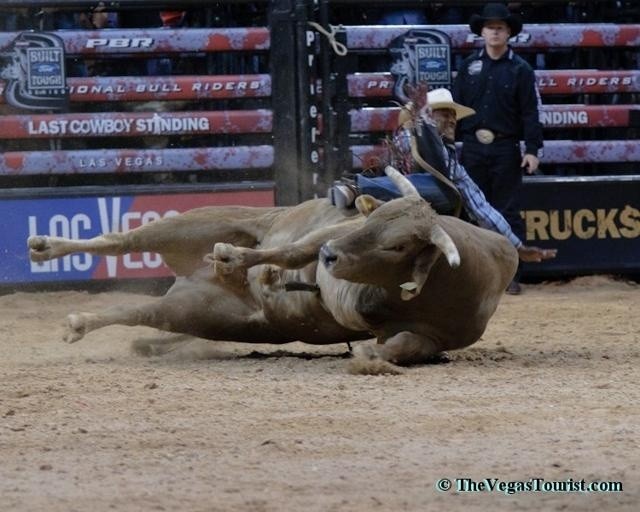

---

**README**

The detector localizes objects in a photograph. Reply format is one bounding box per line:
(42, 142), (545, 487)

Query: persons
(0, 0), (640, 188)
(450, 5), (545, 295)
(328, 88), (558, 262)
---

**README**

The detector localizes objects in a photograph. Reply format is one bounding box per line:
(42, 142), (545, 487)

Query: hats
(399, 88), (477, 128)
(470, 3), (521, 37)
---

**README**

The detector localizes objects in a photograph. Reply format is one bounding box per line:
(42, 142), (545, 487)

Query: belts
(459, 128), (514, 144)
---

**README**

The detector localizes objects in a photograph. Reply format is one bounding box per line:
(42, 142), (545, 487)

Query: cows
(26, 165), (520, 376)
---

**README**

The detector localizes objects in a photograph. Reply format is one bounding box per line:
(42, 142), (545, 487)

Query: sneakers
(328, 185), (357, 211)
